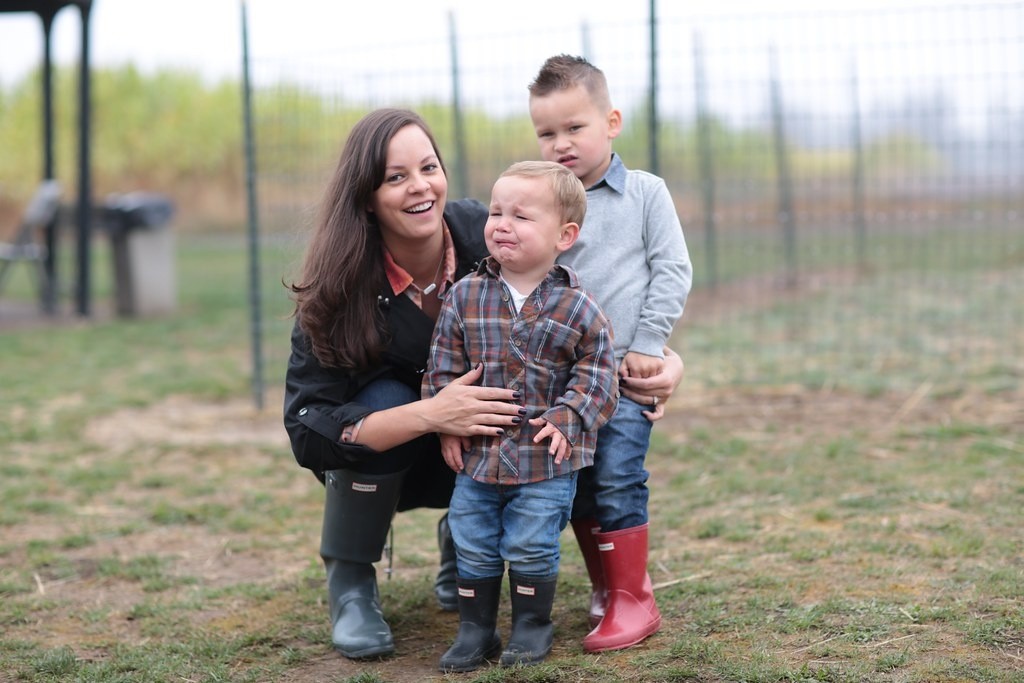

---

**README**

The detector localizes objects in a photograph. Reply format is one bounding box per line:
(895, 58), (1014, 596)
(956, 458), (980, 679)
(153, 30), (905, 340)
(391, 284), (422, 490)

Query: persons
(278, 107), (686, 657)
(419, 161), (621, 674)
(528, 53), (695, 653)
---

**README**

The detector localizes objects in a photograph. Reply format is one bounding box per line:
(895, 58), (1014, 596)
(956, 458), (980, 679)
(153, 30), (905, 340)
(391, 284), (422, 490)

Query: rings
(654, 396), (660, 406)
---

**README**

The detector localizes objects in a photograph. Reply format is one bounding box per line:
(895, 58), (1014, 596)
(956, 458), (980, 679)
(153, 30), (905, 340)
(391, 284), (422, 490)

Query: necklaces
(411, 241), (447, 296)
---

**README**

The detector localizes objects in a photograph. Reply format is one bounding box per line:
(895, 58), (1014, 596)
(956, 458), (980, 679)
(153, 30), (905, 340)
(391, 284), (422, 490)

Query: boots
(499, 566), (557, 667)
(436, 512), (464, 611)
(568, 519), (609, 625)
(319, 466), (398, 660)
(439, 571), (504, 673)
(584, 521), (657, 650)
